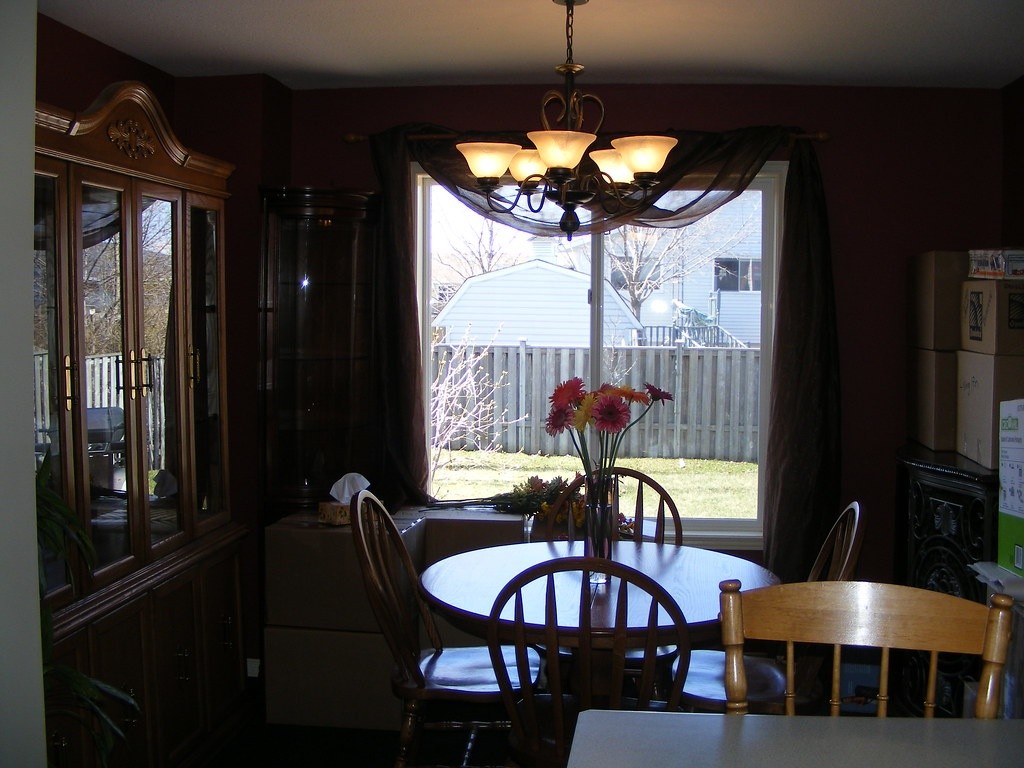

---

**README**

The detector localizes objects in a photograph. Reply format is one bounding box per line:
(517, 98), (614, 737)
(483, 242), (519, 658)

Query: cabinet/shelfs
(262, 498), (526, 732)
(257, 183), (427, 512)
(35, 77), (254, 768)
(890, 436), (999, 718)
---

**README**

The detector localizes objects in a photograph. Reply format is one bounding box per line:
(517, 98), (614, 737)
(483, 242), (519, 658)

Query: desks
(417, 537), (781, 713)
(566, 708), (1024, 768)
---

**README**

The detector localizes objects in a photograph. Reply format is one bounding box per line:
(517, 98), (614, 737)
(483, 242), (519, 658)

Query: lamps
(454, 0), (678, 242)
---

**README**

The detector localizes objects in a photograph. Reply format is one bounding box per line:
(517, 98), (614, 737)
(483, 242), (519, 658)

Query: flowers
(423, 473), (639, 536)
(542, 373), (677, 559)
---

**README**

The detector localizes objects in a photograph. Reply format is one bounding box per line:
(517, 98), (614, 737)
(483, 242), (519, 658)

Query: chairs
(717, 577), (1018, 721)
(344, 466), (868, 768)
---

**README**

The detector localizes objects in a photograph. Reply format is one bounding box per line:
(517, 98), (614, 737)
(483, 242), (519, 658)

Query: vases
(583, 472), (614, 584)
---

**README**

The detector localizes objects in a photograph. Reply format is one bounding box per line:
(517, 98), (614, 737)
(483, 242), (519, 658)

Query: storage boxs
(905, 246), (1024, 577)
